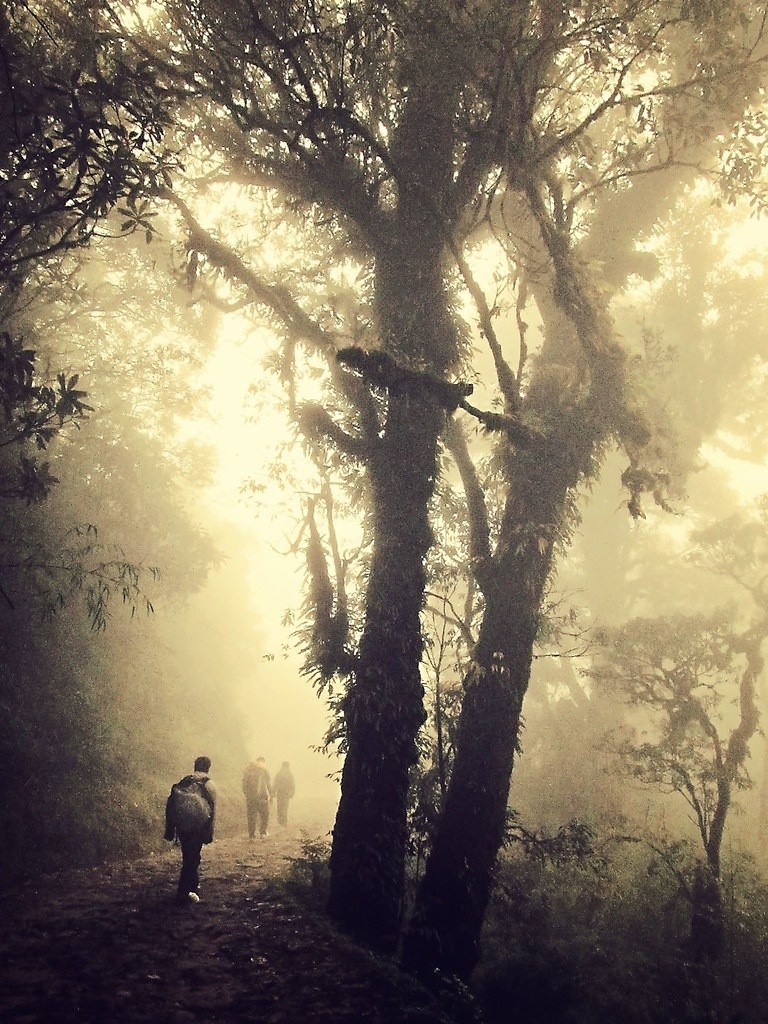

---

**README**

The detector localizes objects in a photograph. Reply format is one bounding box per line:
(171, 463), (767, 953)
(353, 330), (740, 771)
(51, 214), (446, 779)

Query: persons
(241, 757), (273, 840)
(163, 757), (219, 904)
(274, 761), (297, 827)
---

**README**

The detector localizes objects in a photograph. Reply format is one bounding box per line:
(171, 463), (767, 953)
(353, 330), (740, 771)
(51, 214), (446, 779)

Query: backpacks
(171, 776), (214, 831)
(241, 761), (266, 798)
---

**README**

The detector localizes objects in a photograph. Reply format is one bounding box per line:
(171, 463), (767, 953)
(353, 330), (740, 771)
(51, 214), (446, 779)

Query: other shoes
(192, 883), (200, 892)
(180, 891), (199, 906)
(249, 834), (256, 839)
(260, 831), (268, 839)
